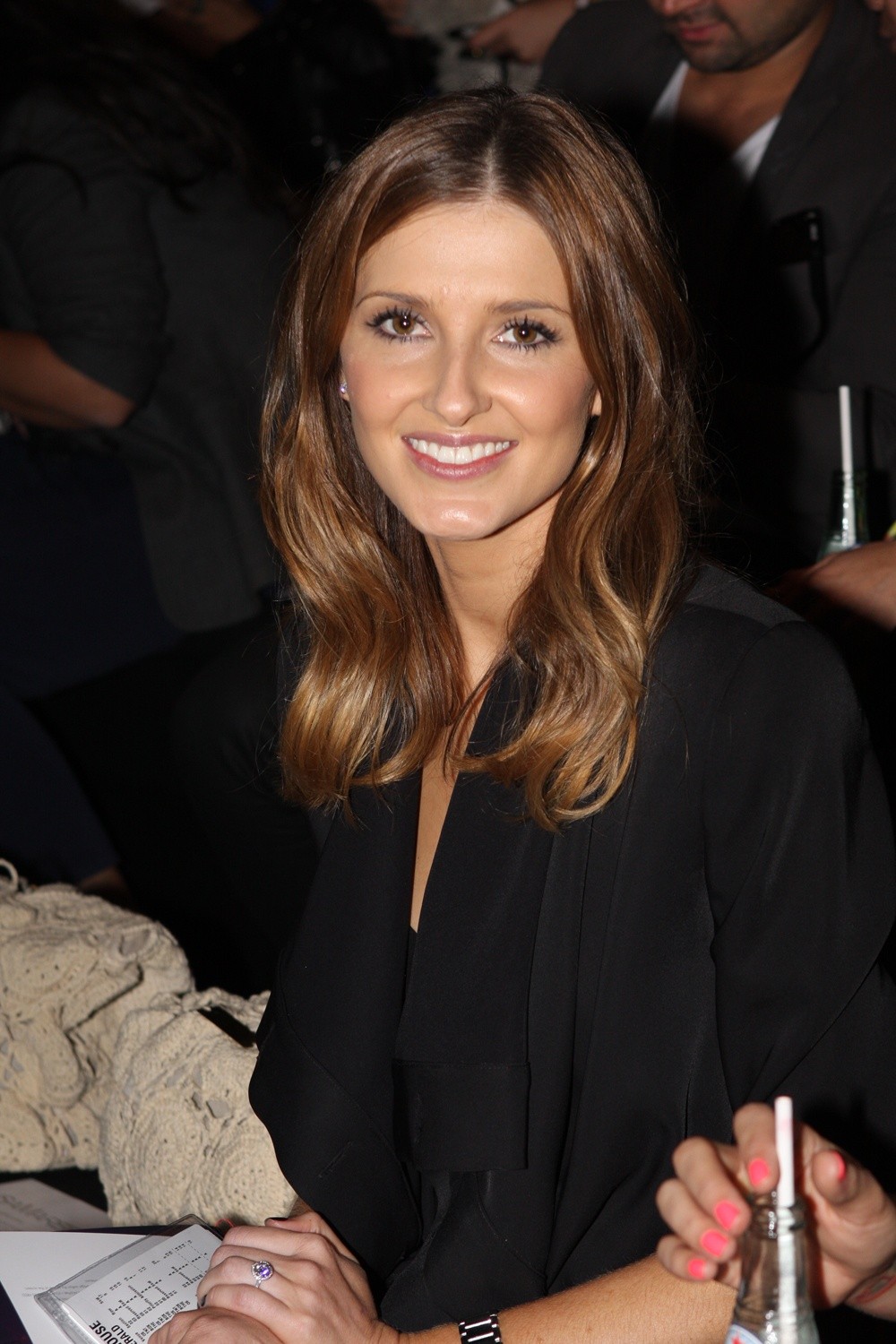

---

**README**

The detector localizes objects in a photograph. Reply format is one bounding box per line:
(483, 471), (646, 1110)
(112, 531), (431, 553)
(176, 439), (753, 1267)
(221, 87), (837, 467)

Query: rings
(249, 1260), (275, 1289)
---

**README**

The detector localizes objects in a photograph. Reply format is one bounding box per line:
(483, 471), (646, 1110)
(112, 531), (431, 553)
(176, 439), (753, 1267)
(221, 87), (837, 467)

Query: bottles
(815, 467), (872, 569)
(720, 1190), (821, 1344)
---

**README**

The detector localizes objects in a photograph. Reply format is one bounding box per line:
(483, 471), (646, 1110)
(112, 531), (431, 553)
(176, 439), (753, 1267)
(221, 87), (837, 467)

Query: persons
(1, 1), (895, 1344)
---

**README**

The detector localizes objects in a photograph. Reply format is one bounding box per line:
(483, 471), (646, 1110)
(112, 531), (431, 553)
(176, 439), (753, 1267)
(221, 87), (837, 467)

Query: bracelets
(458, 1311), (505, 1344)
